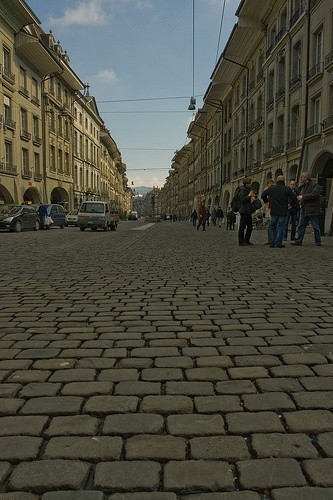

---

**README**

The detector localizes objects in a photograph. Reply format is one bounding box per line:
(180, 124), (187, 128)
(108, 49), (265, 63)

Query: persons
(161, 213), (184, 222)
(226, 210), (235, 230)
(36, 202), (49, 230)
(21, 202), (29, 206)
(191, 210), (197, 226)
(231, 178), (254, 246)
(206, 207), (224, 227)
(197, 201), (207, 231)
(261, 172), (321, 248)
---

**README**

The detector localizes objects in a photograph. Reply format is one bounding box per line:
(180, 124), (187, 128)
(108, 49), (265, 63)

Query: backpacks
(230, 190), (241, 212)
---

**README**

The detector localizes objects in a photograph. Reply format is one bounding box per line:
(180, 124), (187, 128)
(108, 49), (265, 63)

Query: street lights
(188, 95), (224, 198)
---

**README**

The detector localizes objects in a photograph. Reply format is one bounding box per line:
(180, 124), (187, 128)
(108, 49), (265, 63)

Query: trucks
(78, 200), (121, 233)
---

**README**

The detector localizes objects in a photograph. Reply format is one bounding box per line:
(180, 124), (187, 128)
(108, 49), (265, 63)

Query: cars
(128, 211), (139, 221)
(65, 209), (78, 227)
(36, 204), (66, 229)
(0, 203), (40, 231)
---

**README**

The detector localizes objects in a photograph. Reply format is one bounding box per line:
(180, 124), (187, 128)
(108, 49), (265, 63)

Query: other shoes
(290, 241), (302, 246)
(314, 243), (322, 246)
(267, 242), (286, 248)
(238, 241), (253, 246)
